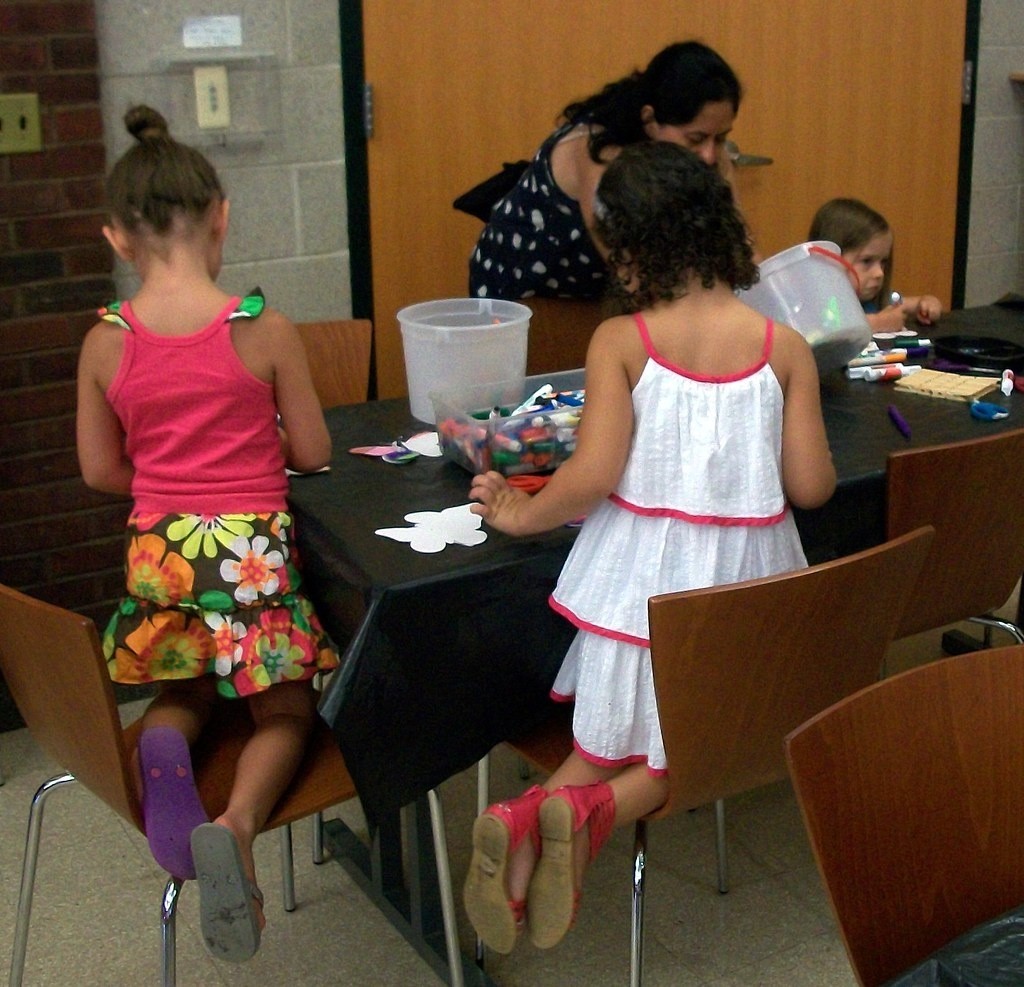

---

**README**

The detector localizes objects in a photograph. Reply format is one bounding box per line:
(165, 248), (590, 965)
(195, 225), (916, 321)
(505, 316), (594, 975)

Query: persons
(459, 33), (747, 313)
(811, 189), (944, 339)
(449, 144), (844, 965)
(76, 98), (339, 960)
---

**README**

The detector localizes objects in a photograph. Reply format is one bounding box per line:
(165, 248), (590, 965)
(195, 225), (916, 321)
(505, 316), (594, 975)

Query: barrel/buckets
(394, 298), (531, 425)
(734, 239), (870, 377)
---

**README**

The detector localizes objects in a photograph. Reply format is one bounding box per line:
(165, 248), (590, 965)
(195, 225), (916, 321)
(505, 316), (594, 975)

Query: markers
(439, 382), (588, 474)
(891, 291), (902, 309)
(847, 346), (929, 382)
(886, 404), (913, 440)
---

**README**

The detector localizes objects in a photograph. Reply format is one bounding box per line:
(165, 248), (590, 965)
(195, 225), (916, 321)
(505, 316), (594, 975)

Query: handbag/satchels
(453, 159), (533, 224)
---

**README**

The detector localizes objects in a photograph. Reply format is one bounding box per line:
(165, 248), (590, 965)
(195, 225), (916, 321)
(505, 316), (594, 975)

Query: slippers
(189, 822), (265, 963)
(141, 725), (209, 881)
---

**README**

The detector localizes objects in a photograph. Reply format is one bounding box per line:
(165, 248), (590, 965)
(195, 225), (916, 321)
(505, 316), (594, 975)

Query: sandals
(463, 780), (616, 955)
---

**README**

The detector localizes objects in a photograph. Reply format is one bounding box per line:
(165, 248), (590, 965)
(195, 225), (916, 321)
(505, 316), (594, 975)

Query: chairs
(472, 523), (940, 987)
(0, 584), (465, 987)
(884, 428), (1024, 649)
(296, 317), (375, 414)
(781, 646), (1024, 985)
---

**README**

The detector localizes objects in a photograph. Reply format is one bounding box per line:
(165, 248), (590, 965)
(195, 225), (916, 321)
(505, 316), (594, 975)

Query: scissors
(508, 475), (552, 494)
(965, 396), (1010, 421)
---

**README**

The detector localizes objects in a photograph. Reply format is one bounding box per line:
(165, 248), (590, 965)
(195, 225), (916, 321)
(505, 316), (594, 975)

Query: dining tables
(285, 291), (1022, 987)
